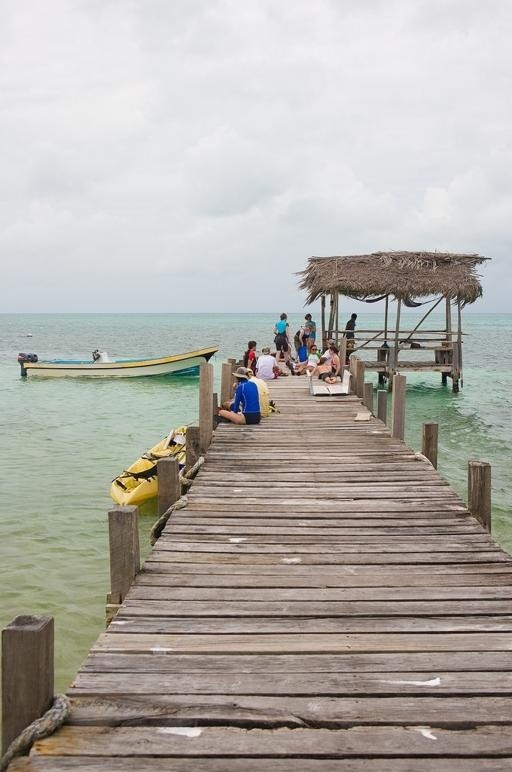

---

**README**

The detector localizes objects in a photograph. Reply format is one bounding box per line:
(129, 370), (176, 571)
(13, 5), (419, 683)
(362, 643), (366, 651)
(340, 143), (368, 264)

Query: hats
(232, 367), (250, 381)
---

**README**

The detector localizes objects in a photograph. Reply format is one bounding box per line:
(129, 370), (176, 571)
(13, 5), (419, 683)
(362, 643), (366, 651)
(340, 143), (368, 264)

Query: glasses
(311, 348), (317, 351)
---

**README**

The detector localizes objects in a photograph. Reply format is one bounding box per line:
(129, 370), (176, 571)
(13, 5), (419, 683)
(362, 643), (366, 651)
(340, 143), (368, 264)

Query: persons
(92, 349), (109, 363)
(218, 312), (356, 425)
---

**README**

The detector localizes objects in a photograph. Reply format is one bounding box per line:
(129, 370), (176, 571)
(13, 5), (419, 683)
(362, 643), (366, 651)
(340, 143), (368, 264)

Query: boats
(15, 344), (220, 378)
(106, 419), (199, 508)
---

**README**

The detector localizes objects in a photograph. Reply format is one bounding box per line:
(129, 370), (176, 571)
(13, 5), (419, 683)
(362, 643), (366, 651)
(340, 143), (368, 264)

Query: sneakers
(286, 359), (301, 376)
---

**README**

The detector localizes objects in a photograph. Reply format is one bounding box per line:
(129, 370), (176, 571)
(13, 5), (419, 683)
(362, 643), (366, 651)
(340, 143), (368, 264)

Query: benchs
(398, 347), (453, 364)
(346, 347), (395, 368)
